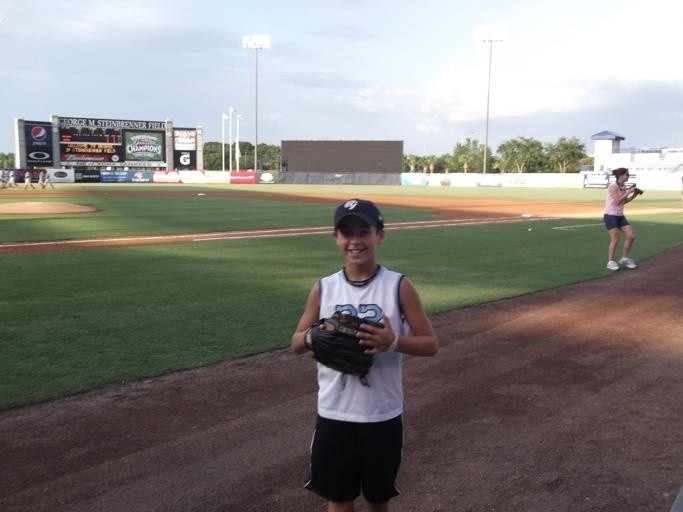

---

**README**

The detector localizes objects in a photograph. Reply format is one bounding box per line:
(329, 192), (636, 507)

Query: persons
(603, 167), (639, 271)
(292, 199), (439, 512)
(0, 167), (55, 190)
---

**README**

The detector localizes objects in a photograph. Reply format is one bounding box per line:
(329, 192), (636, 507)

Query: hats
(335, 199), (384, 228)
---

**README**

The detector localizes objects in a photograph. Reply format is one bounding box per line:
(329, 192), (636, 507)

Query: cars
(580, 165), (593, 173)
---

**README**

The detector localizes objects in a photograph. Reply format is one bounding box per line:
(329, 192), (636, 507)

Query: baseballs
(528, 227), (532, 232)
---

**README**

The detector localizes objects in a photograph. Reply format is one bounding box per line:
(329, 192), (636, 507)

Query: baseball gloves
(303, 312), (384, 377)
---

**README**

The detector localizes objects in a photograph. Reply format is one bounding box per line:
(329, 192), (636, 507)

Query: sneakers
(607, 257), (638, 271)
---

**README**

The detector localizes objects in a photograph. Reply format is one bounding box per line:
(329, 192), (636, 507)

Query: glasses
(623, 173), (629, 176)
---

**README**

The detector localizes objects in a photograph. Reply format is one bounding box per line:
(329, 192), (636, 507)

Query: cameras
(631, 184), (643, 195)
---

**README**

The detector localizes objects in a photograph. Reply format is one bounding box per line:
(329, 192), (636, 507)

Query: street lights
(483, 40), (503, 174)
(243, 35), (271, 171)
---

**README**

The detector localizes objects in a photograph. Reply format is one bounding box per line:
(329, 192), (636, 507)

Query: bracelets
(387, 333), (400, 356)
